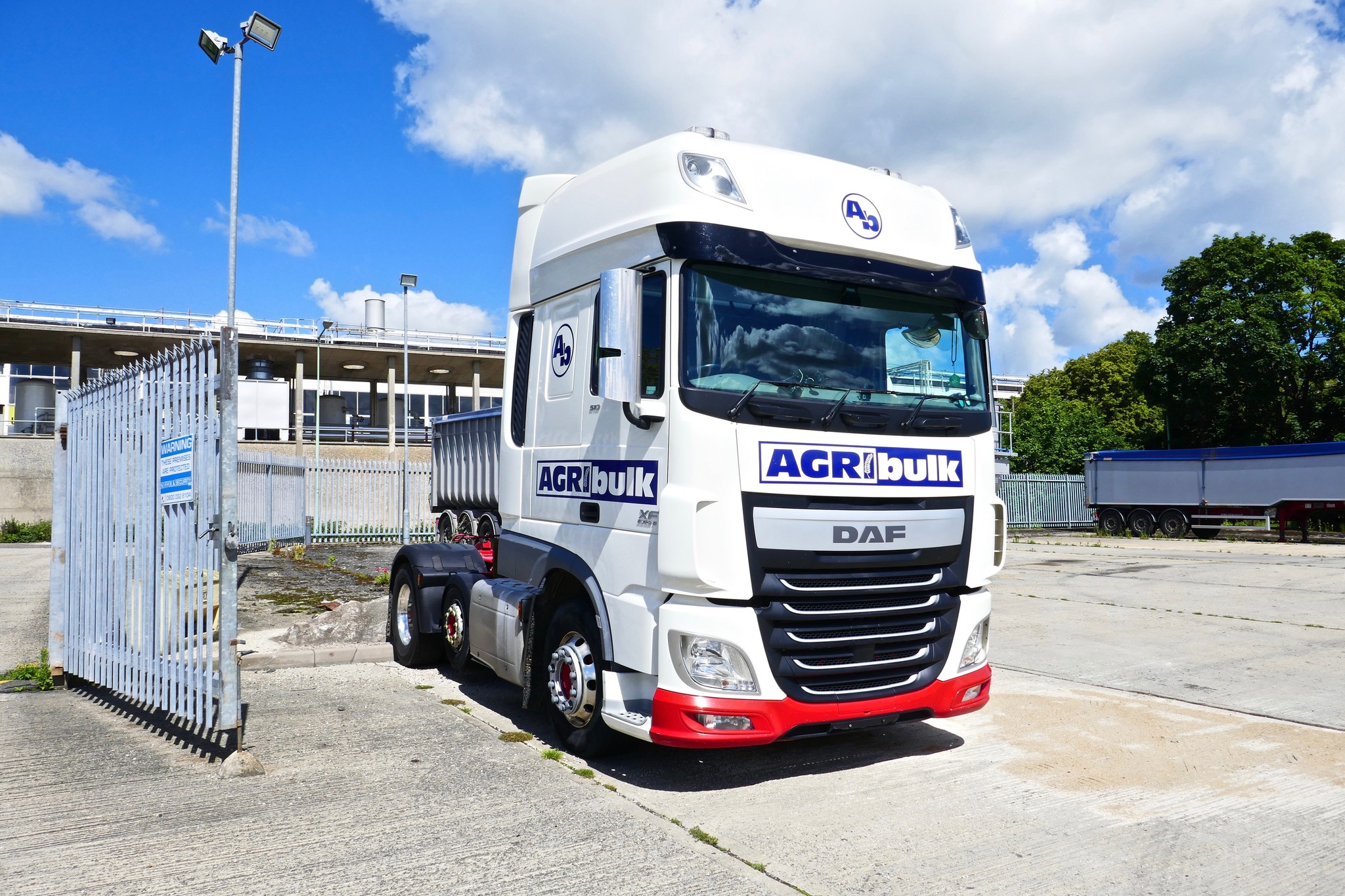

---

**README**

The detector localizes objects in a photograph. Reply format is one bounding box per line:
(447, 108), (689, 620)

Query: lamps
(427, 365), (455, 374)
(106, 317), (116, 326)
(110, 345), (142, 356)
(339, 359), (369, 369)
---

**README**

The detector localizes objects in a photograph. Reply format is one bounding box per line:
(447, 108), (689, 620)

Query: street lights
(197, 11), (282, 326)
(398, 273), (418, 546)
(314, 320), (335, 547)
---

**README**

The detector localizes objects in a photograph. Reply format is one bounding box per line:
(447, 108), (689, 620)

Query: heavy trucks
(1081, 439), (1345, 540)
(428, 406), (502, 571)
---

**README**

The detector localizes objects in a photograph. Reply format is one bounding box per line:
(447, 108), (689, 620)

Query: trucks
(387, 125), (1007, 755)
